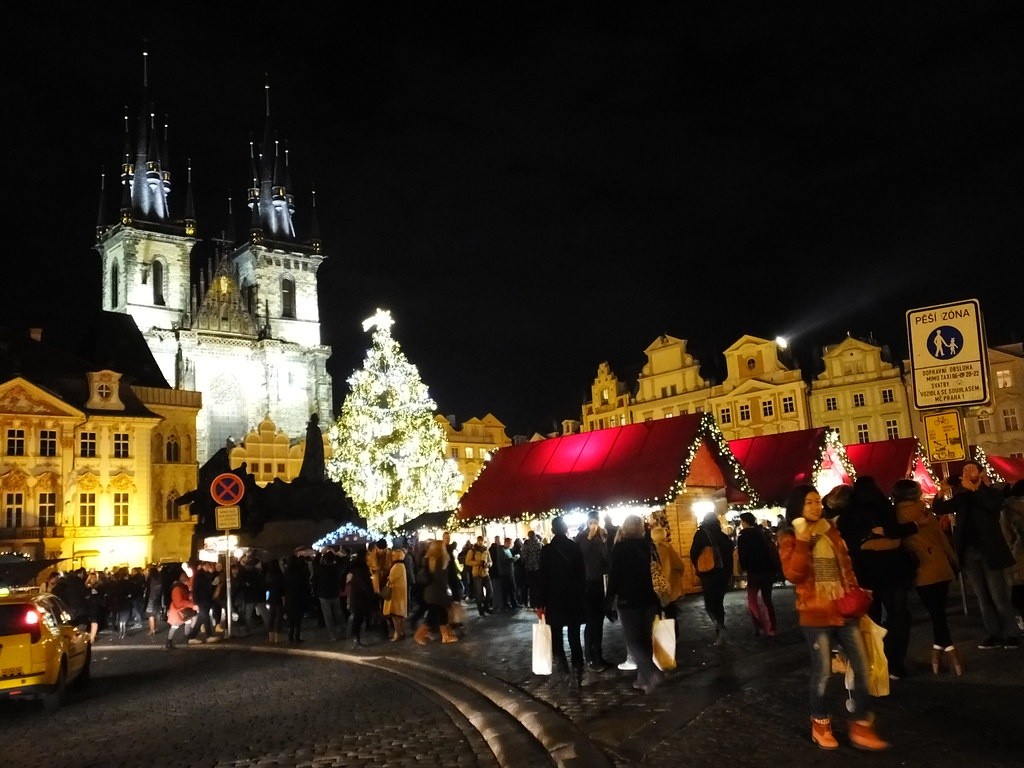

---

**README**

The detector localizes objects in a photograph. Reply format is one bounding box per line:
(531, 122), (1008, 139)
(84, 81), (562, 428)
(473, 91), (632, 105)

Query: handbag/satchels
(845, 614), (889, 696)
(531, 613), (552, 675)
(447, 601), (465, 623)
(652, 610), (677, 672)
(651, 542), (670, 598)
(768, 537), (784, 581)
(694, 525), (724, 577)
(382, 584), (393, 601)
(178, 608), (196, 621)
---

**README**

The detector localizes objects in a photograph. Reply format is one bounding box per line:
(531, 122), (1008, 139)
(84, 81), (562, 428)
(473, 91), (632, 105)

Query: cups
(791, 517), (808, 534)
(590, 524), (597, 531)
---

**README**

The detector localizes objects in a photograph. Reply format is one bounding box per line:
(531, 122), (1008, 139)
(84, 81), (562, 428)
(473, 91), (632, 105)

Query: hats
(294, 546), (304, 553)
(174, 568), (184, 580)
(892, 480), (924, 500)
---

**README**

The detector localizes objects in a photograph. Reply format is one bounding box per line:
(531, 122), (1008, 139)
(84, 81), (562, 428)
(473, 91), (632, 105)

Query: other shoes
(997, 636), (1019, 650)
(88, 618), (394, 650)
(978, 636), (1003, 649)
(478, 602), (777, 696)
(1017, 616), (1024, 630)
(891, 667), (905, 680)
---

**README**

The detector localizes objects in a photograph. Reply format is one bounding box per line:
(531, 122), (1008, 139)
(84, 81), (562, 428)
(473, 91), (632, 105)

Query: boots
(390, 616), (406, 642)
(849, 719), (890, 752)
(931, 643), (944, 675)
(944, 643), (965, 676)
(811, 715), (839, 751)
(439, 624), (461, 643)
(413, 623), (431, 645)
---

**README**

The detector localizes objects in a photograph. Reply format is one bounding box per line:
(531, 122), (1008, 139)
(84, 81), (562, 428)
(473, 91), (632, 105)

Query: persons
(688, 511), (734, 646)
(300, 413), (324, 477)
(40, 531), (546, 650)
(933, 463), (1024, 651)
(778, 487), (888, 751)
(651, 527), (684, 643)
(604, 517), (663, 691)
(739, 513), (780, 639)
(825, 475), (964, 680)
(535, 510), (621, 690)
(1001, 479), (1023, 631)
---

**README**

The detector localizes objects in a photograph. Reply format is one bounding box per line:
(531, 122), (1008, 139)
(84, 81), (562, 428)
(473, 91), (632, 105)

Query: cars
(0, 592), (93, 712)
